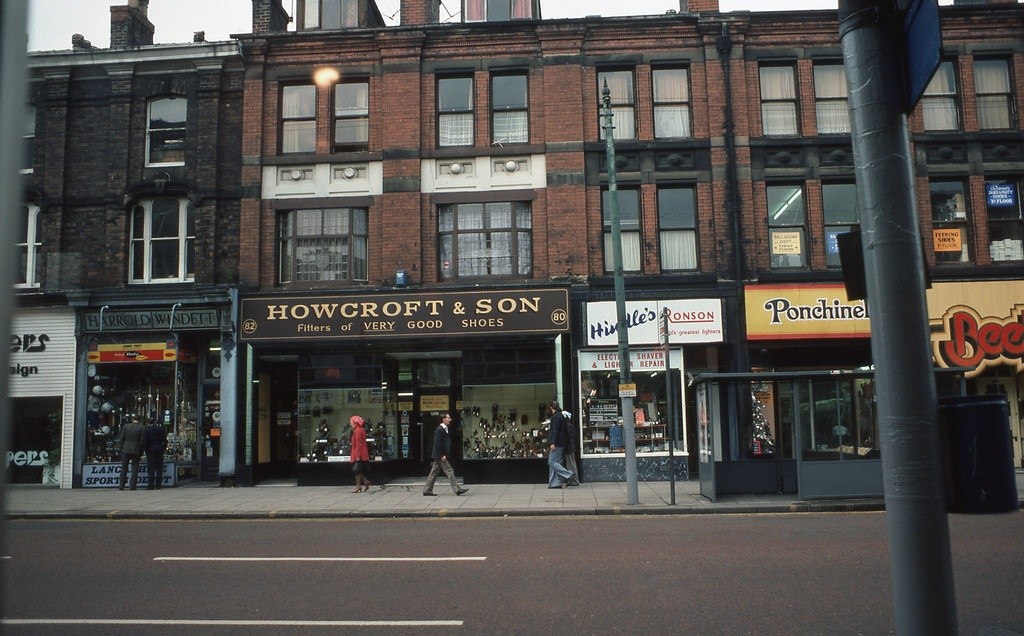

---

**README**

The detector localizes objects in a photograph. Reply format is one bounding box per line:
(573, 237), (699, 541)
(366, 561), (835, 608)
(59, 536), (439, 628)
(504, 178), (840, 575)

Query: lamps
(168, 303), (181, 339)
(773, 188), (802, 221)
(219, 310), (236, 363)
(96, 306), (109, 342)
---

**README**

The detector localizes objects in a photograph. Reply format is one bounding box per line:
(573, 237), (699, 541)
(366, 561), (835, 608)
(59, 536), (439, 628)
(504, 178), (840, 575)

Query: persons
(143, 417), (168, 490)
(423, 413), (469, 496)
(118, 416), (145, 490)
(350, 416), (371, 492)
(561, 411), (580, 486)
(547, 401), (576, 488)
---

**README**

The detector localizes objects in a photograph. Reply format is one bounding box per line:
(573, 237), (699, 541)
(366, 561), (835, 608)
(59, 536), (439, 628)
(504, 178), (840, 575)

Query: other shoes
(463, 405), (481, 417)
(130, 488), (136, 490)
(568, 484), (579, 486)
(119, 487), (124, 490)
(156, 487), (161, 490)
(147, 488), (154, 490)
(456, 488), (470, 496)
(343, 423), (350, 433)
(479, 417), (488, 429)
(565, 473), (576, 488)
(548, 486), (562, 489)
(423, 492), (438, 497)
(312, 406), (321, 418)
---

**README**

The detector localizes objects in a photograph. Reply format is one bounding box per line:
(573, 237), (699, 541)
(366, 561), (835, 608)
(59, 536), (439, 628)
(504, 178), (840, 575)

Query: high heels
(351, 486), (362, 493)
(364, 481), (373, 491)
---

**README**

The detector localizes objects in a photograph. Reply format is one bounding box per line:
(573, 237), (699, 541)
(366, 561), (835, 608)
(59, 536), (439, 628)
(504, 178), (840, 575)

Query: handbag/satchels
(352, 460), (365, 475)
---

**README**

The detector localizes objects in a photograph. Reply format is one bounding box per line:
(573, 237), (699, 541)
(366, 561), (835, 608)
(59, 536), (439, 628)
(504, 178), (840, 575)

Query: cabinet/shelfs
(583, 423), (669, 453)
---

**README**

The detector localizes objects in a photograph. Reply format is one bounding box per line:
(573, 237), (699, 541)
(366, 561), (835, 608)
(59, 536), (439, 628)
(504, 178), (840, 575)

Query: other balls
(102, 402), (111, 412)
(102, 426), (110, 434)
(93, 386), (102, 395)
